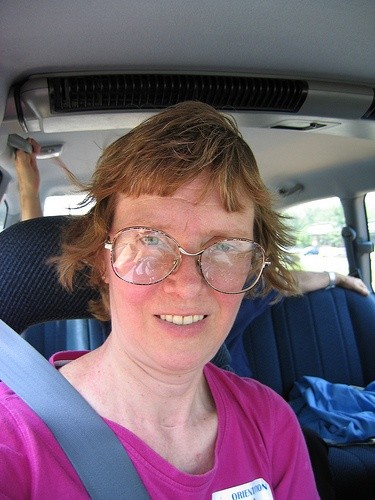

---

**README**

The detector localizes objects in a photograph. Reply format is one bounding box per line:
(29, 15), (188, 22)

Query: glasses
(103, 227), (272, 294)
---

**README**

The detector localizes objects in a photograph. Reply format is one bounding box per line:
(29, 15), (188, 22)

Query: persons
(0, 101), (318, 500)
(14, 137), (42, 221)
(225, 269), (370, 378)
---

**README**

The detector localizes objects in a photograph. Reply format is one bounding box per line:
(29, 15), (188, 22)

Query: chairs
(0, 216), (375, 500)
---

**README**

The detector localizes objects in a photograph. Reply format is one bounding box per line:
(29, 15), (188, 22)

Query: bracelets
(328, 272), (336, 285)
